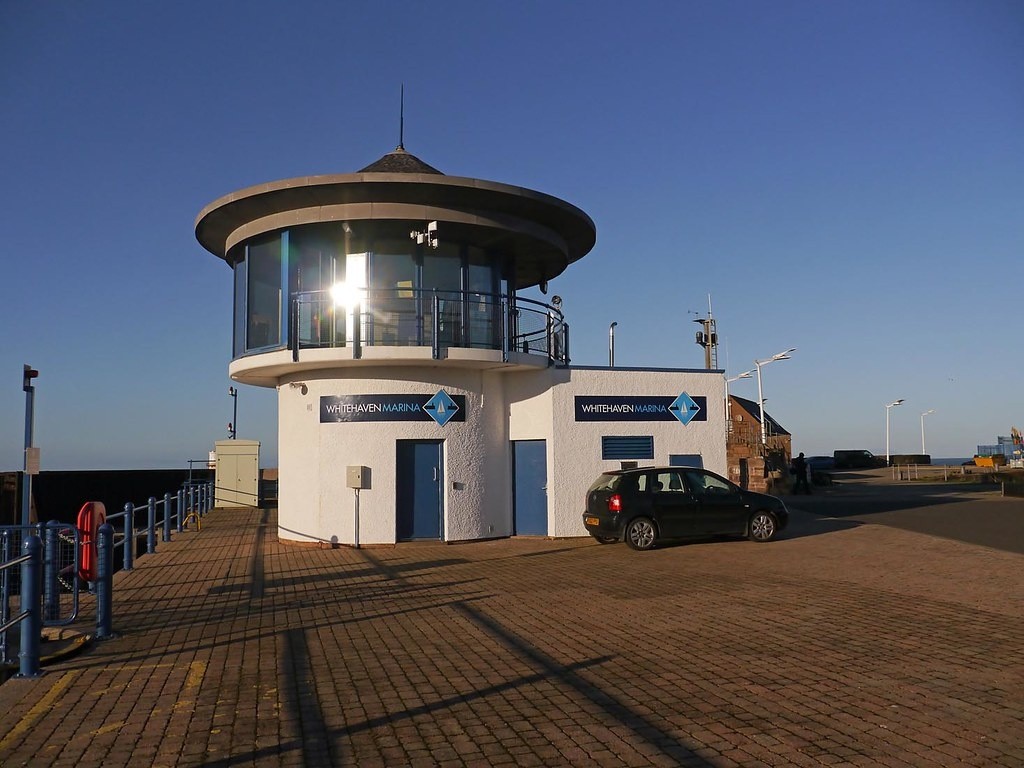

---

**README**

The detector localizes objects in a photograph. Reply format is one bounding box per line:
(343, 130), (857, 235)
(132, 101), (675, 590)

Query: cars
(804, 456), (834, 470)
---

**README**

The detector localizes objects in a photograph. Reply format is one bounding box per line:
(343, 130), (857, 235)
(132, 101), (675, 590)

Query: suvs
(582, 466), (790, 551)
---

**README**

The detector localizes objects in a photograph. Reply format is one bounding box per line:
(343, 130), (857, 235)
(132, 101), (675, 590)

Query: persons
(792, 452), (813, 493)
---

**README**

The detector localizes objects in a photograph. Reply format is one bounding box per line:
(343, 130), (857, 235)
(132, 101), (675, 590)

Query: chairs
(669, 480), (682, 491)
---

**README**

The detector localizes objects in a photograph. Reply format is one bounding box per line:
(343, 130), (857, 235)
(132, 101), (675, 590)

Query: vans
(834, 450), (886, 468)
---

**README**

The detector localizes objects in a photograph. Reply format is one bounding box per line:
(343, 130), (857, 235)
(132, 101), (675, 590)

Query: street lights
(756, 348), (796, 445)
(920, 410), (937, 455)
(886, 399), (906, 467)
(725, 368), (757, 422)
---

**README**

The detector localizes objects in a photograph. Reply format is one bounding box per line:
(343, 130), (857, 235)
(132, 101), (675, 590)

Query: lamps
(552, 296), (562, 310)
(289, 381), (306, 393)
